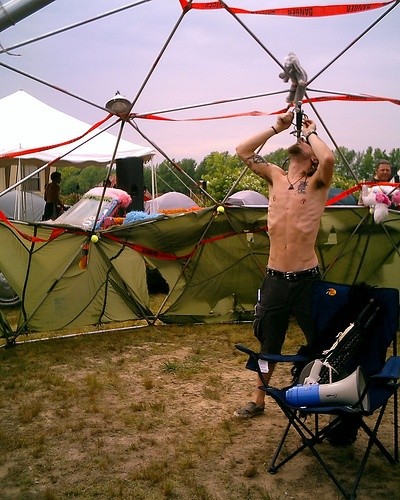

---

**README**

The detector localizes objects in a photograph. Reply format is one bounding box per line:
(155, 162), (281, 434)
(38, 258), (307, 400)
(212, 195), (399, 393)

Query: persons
(44, 172), (67, 221)
(236, 111), (336, 419)
(371, 159), (400, 183)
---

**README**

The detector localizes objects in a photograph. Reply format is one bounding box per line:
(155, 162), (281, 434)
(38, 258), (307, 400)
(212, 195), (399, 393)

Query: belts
(266, 266), (319, 280)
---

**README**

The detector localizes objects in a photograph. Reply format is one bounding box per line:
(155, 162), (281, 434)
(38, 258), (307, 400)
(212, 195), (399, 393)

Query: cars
(325, 187), (358, 233)
(0, 189), (47, 310)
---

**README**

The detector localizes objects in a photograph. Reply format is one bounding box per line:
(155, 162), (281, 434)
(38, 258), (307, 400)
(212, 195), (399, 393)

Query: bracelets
(306, 131), (317, 140)
(272, 126), (278, 134)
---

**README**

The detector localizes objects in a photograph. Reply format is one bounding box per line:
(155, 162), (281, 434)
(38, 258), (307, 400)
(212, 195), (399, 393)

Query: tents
(144, 191), (199, 215)
(226, 190), (269, 205)
(0, 90), (158, 220)
(0, 187), (46, 222)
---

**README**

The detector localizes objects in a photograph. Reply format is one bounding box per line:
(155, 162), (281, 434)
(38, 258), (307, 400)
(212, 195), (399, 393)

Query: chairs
(235, 280), (400, 500)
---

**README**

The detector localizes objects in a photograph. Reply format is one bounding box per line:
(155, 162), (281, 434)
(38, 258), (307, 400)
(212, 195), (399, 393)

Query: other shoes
(234, 400), (265, 418)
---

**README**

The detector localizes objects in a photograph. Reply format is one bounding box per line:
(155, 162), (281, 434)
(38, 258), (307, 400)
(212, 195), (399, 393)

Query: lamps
(105, 91), (132, 115)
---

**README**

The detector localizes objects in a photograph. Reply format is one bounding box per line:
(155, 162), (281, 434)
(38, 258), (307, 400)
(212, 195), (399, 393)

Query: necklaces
(286, 171), (304, 189)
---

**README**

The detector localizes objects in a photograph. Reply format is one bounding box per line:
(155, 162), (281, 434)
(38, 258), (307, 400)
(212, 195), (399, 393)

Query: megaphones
(282, 366), (373, 413)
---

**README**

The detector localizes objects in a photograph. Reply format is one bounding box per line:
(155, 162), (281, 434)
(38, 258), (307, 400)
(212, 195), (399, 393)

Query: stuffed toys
(362, 184), (400, 224)
(279, 52), (308, 104)
